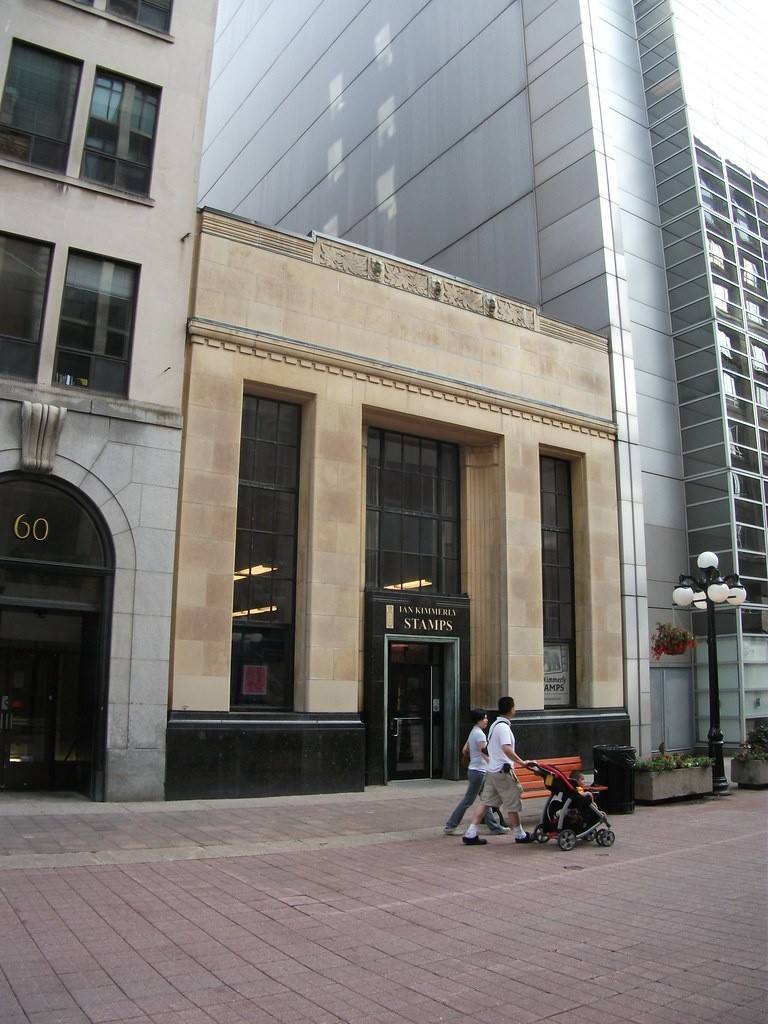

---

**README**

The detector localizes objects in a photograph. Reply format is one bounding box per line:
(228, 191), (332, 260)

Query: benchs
(479, 756), (608, 829)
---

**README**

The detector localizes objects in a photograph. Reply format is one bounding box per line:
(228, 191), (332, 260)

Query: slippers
(463, 835), (488, 845)
(515, 832), (539, 843)
(444, 827), (463, 836)
(491, 827), (511, 834)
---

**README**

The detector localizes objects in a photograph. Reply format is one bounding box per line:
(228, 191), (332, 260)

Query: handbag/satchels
(462, 750), (471, 766)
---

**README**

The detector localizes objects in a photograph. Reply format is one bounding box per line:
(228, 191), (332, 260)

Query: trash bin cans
(594, 744), (637, 814)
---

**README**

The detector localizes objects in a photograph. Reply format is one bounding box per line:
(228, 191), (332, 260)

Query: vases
(662, 640), (687, 655)
(634, 765), (713, 806)
(731, 759), (768, 790)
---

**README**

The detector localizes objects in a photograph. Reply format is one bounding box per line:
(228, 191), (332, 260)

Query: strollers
(521, 761), (615, 852)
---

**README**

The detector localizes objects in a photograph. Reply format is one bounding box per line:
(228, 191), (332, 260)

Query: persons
(569, 771), (584, 794)
(444, 697), (539, 845)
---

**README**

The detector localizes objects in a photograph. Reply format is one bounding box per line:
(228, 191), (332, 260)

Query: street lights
(671, 548), (747, 795)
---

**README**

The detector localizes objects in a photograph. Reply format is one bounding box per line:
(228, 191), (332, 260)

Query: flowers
(650, 622), (698, 662)
(634, 752), (715, 773)
(732, 721), (768, 760)
